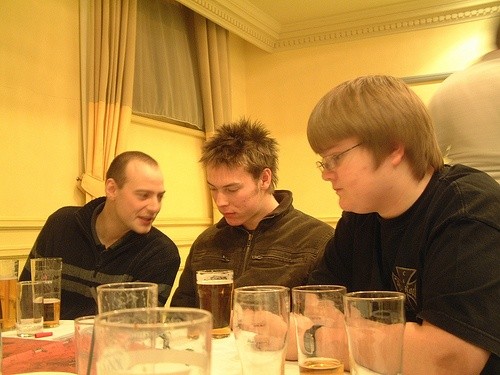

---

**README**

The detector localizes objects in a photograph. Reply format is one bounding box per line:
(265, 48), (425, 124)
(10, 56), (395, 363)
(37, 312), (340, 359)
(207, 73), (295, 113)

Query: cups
(30, 257), (63, 328)
(231, 285), (290, 375)
(291, 284), (346, 375)
(343, 290), (405, 375)
(196, 269), (234, 339)
(93, 307), (212, 375)
(74, 314), (96, 375)
(96, 282), (158, 314)
(15, 282), (44, 337)
(0, 259), (20, 332)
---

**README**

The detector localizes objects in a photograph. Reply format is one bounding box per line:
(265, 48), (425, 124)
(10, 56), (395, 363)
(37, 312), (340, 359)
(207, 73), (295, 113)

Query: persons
(425, 15), (500, 182)
(164, 118), (336, 324)
(18, 151), (181, 320)
(230, 74), (499, 374)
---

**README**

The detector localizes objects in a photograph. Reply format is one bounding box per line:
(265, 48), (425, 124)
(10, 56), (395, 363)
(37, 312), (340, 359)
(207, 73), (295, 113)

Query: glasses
(314, 141), (366, 172)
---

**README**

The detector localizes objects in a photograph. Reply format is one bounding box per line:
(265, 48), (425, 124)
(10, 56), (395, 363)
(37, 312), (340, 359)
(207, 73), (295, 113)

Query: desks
(0, 319), (382, 375)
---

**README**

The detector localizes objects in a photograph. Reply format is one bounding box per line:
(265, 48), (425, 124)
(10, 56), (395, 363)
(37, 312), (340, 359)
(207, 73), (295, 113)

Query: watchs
(303, 325), (324, 358)
